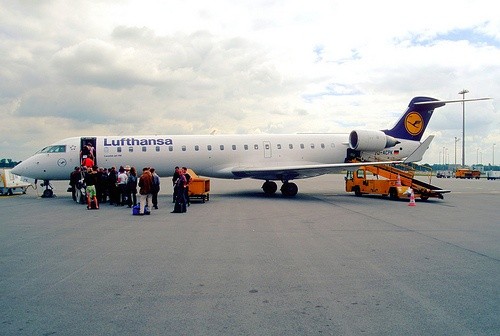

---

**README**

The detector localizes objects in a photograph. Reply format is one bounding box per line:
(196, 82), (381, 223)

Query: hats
(124, 165), (131, 170)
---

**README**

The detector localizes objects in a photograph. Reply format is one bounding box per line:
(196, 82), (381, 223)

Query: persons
(84, 168), (100, 209)
(182, 167), (192, 207)
(115, 164), (133, 206)
(127, 167), (137, 208)
(81, 139), (96, 160)
(170, 168), (187, 213)
(97, 166), (120, 206)
(172, 167), (180, 203)
(69, 165), (99, 204)
(82, 155), (95, 168)
(148, 167), (160, 209)
(137, 167), (152, 215)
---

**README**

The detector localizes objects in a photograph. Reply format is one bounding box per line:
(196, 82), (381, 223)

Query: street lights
(493, 144), (497, 166)
(455, 137), (460, 166)
(480, 151), (482, 164)
(458, 88), (469, 167)
(443, 147), (446, 171)
(477, 148), (479, 164)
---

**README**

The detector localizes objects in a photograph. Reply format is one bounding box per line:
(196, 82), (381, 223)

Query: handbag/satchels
(90, 200), (100, 208)
(133, 204), (150, 215)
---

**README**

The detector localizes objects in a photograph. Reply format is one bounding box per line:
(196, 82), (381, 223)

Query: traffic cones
(395, 172), (402, 187)
(407, 189), (416, 207)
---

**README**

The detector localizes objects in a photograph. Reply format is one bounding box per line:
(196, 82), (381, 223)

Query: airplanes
(9, 96), (496, 198)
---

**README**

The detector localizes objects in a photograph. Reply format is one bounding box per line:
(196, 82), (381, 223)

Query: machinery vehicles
(344, 164), (451, 202)
